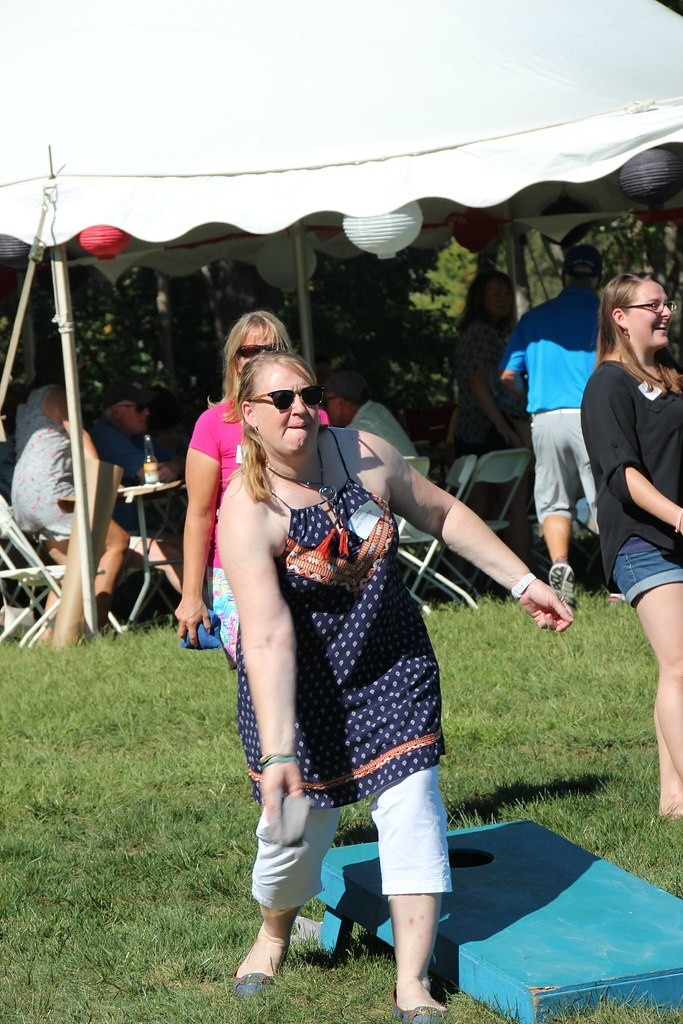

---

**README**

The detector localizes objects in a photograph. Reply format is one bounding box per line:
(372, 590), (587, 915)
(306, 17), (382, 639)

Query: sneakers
(607, 593), (625, 606)
(549, 561), (574, 604)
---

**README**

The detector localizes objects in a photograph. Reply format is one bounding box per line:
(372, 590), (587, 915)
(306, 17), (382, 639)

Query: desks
(114, 480), (186, 631)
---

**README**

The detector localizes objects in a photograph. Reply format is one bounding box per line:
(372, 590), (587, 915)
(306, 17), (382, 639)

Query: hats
(103, 382), (158, 407)
(321, 371), (368, 402)
(564, 244), (603, 277)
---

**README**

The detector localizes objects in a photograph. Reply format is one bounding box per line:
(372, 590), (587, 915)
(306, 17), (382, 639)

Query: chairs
(0, 493), (126, 648)
(388, 403), (592, 612)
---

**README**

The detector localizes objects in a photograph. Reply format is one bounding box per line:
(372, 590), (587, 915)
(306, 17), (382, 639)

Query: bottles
(143, 435), (160, 484)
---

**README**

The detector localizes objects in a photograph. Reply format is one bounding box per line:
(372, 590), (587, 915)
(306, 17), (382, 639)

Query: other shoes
(392, 985), (450, 1024)
(232, 937), (290, 999)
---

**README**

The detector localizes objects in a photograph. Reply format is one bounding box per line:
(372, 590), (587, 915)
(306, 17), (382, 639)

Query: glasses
(320, 395), (337, 408)
(242, 385), (324, 410)
(613, 299), (677, 313)
(116, 403), (148, 413)
(235, 343), (285, 358)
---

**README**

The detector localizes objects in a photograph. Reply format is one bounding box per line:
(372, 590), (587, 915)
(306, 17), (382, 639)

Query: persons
(322, 367), (428, 473)
(498, 245), (628, 605)
(173, 311), (330, 666)
(580, 272), (683, 818)
(453, 270), (535, 581)
(219, 351), (575, 1024)
(12, 339), (131, 645)
(88, 381), (187, 594)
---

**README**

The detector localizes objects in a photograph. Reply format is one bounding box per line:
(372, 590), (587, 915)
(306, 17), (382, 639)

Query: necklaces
(263, 445), (337, 501)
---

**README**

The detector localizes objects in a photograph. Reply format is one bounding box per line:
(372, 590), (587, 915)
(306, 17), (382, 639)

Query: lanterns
(0, 234), (31, 271)
(618, 148), (683, 210)
(77, 225), (131, 260)
(342, 201), (423, 260)
(452, 211), (497, 253)
(256, 237), (317, 292)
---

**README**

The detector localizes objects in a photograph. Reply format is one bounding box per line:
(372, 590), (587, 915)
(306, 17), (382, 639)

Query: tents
(0, 0), (683, 633)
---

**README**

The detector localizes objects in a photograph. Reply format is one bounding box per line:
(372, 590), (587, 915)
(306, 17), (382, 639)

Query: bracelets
(675, 509), (683, 533)
(257, 754), (300, 769)
(511, 573), (537, 599)
